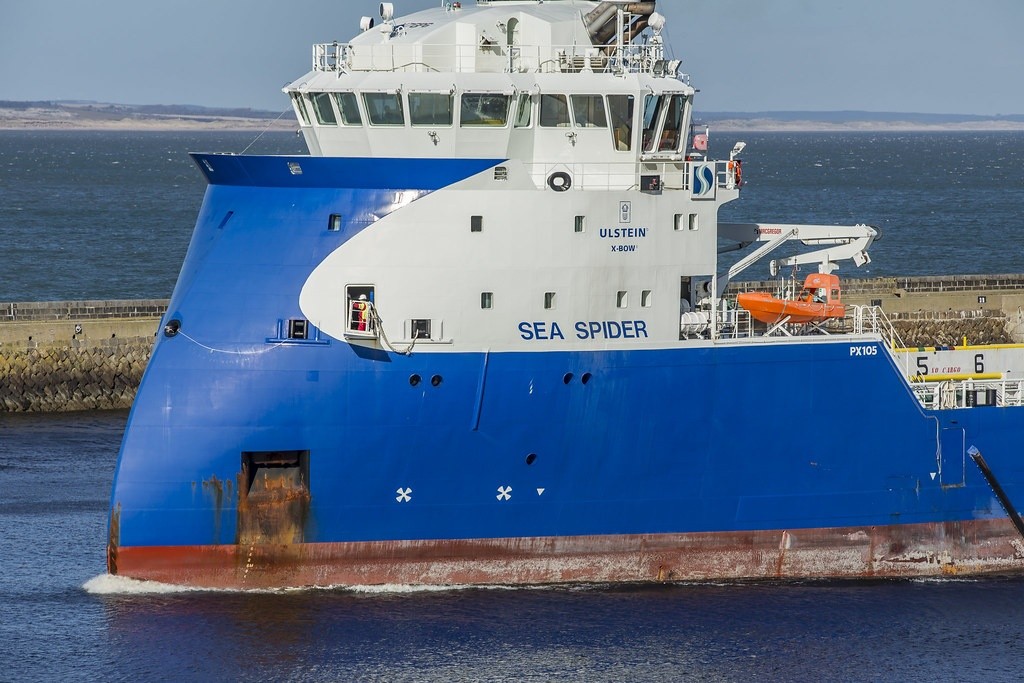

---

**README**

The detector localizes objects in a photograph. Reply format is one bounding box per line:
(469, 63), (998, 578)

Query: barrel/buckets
(966, 389), (977, 408)
(986, 388), (996, 406)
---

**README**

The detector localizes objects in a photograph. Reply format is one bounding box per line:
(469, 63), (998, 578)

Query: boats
(104, 0), (1024, 589)
(738, 273), (844, 325)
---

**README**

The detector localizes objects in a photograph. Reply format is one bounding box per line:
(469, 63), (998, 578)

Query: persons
(353, 294), (367, 331)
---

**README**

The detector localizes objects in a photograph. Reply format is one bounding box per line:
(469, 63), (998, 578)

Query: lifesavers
(548, 171), (572, 192)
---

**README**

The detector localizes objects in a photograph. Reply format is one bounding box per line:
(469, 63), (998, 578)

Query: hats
(358, 294), (367, 300)
